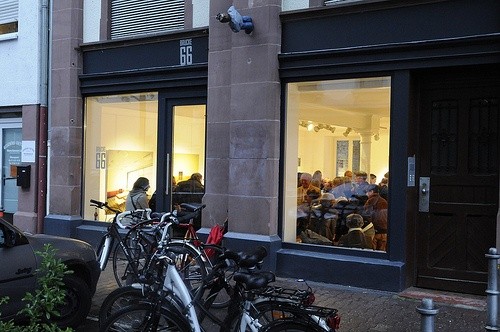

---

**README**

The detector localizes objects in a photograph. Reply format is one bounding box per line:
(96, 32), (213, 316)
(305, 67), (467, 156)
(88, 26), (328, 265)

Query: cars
(0, 219), (99, 329)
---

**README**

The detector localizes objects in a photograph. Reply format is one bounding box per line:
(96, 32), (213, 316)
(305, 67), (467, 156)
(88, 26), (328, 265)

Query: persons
(148, 172), (205, 237)
(121, 177), (150, 280)
(298, 168), (389, 252)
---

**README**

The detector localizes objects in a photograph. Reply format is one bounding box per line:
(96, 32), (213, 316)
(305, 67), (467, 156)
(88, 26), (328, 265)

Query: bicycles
(89, 198), (341, 332)
